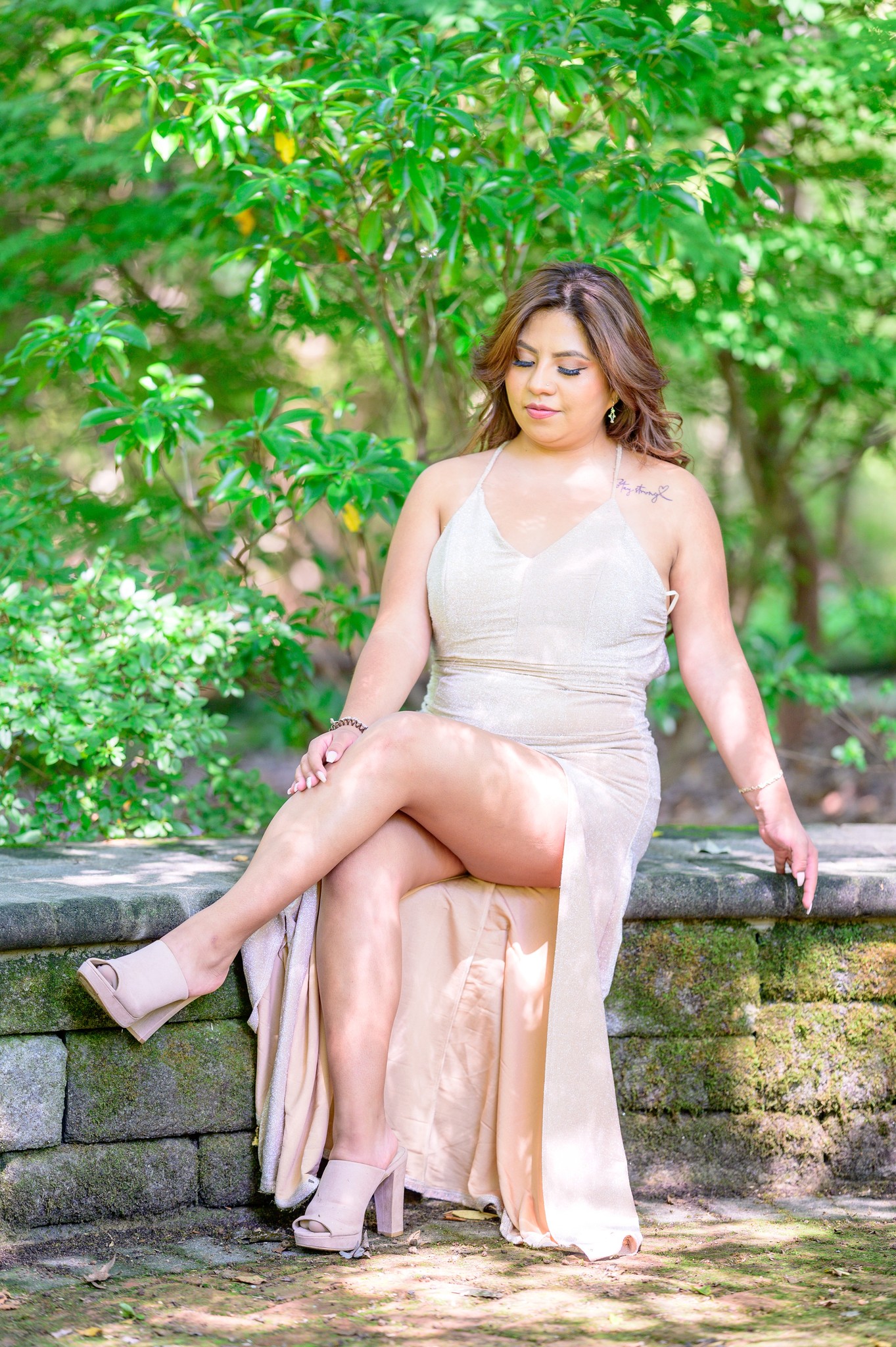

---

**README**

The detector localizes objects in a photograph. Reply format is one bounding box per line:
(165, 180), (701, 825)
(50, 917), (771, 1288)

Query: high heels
(292, 1146), (408, 1252)
(76, 938), (200, 1044)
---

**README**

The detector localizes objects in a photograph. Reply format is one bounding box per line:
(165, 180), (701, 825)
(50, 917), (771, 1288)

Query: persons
(76, 259), (824, 1256)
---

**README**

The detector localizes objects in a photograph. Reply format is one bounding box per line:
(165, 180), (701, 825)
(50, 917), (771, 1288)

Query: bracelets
(738, 769), (784, 794)
(327, 716), (368, 734)
